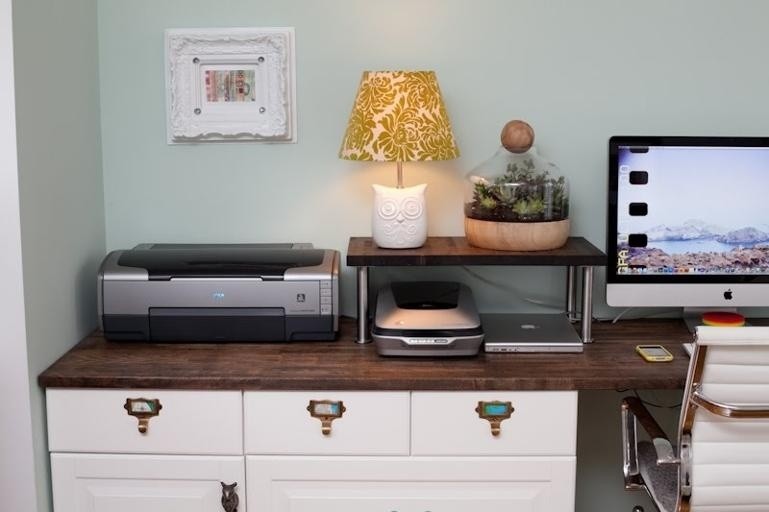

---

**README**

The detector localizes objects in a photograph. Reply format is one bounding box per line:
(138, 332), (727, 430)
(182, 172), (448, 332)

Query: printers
(96, 242), (342, 343)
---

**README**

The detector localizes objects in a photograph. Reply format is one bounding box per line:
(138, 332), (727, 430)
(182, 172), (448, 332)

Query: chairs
(619, 326), (769, 512)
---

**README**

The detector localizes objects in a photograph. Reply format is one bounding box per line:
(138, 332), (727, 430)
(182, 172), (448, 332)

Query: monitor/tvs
(606, 135), (769, 333)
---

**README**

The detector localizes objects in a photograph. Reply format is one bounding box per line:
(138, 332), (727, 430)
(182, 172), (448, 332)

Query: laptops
(480, 313), (584, 354)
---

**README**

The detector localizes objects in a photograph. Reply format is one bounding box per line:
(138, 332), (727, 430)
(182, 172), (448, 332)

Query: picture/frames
(160, 27), (299, 149)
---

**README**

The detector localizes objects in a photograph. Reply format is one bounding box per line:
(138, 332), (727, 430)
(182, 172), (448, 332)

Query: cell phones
(637, 344), (675, 363)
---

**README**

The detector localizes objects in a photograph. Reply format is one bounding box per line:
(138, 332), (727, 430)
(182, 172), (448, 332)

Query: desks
(40, 311), (768, 512)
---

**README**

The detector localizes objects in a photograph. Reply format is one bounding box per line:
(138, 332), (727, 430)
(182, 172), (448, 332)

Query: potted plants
(463, 144), (575, 250)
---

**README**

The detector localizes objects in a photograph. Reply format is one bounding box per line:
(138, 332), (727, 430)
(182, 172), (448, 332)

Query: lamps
(338, 64), (459, 253)
